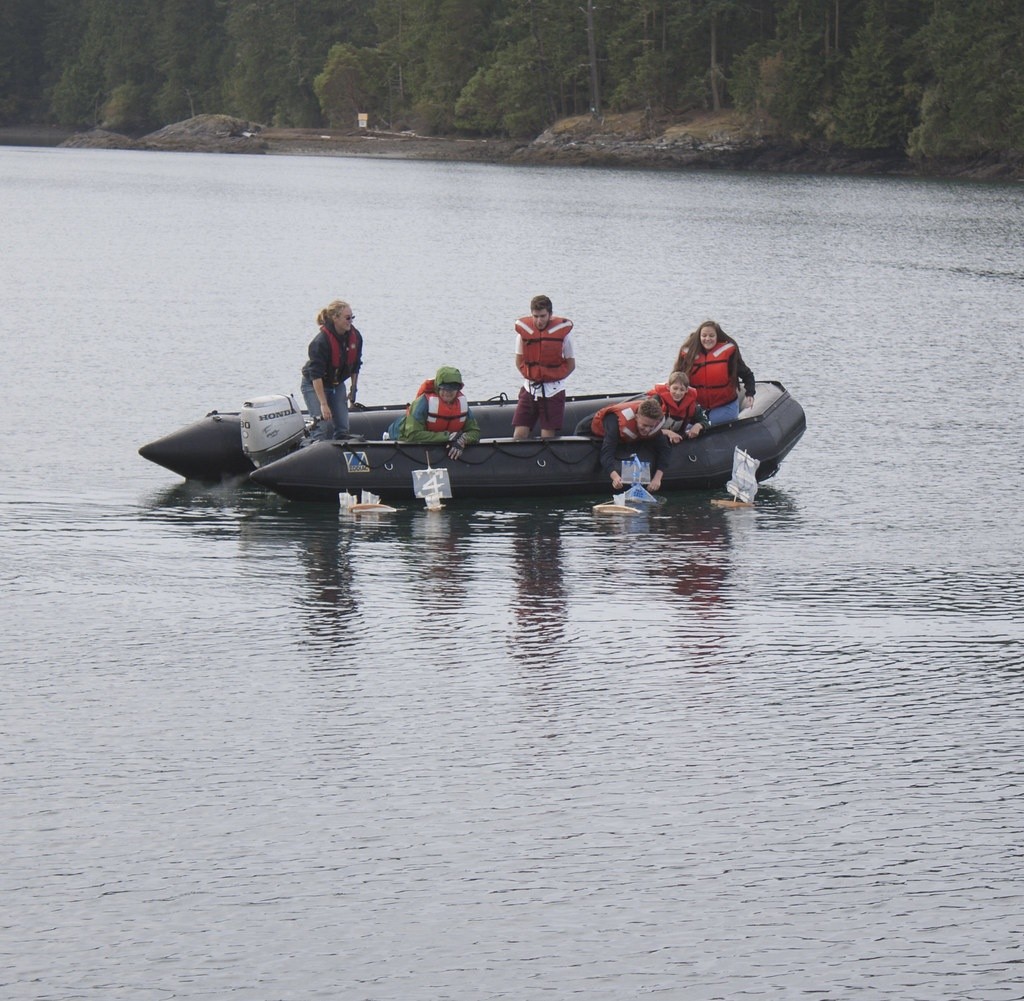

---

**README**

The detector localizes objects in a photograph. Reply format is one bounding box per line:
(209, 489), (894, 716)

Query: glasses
(337, 315), (355, 321)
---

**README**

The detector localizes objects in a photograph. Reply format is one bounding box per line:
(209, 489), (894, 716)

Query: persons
(383, 366), (481, 459)
(301, 303), (363, 441)
(574, 401), (672, 491)
(648, 372), (709, 444)
(513, 295), (575, 437)
(674, 320), (755, 425)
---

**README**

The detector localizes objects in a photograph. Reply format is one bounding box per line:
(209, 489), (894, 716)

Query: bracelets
(350, 386), (357, 392)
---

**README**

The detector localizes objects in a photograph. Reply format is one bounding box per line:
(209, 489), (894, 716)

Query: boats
(138, 380), (808, 508)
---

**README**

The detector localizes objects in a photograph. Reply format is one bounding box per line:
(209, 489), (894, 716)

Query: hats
(438, 383), (461, 390)
